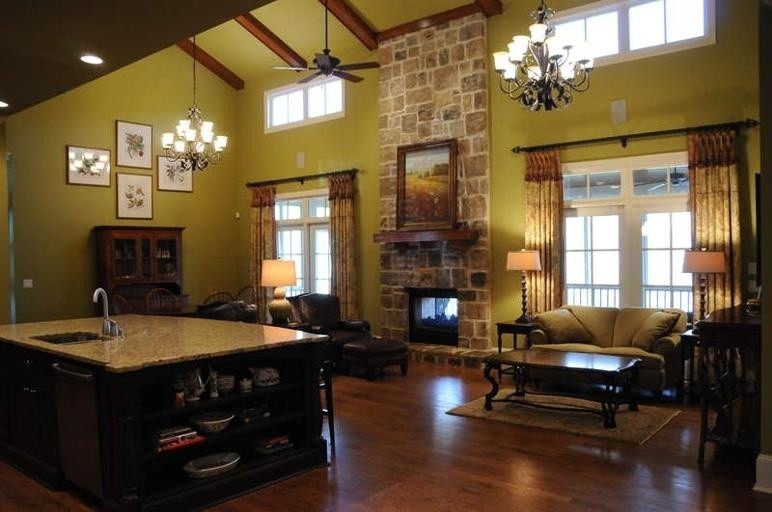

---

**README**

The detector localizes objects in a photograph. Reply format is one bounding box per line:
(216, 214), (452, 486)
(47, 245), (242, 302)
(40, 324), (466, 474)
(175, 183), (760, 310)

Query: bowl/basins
(183, 452), (242, 478)
(187, 411), (235, 435)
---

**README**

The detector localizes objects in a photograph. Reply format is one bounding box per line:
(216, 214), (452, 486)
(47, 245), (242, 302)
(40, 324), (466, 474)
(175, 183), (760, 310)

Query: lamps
(506, 248), (542, 323)
(683, 248), (727, 327)
(160, 28), (228, 172)
(492, 0), (594, 113)
(261, 256), (296, 324)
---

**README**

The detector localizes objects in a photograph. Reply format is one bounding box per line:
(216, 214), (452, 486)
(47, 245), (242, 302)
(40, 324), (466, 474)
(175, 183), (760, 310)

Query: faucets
(93, 288), (111, 335)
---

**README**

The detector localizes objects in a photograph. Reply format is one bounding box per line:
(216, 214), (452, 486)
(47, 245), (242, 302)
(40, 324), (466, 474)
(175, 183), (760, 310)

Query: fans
(633, 167), (689, 191)
(272, 0), (380, 83)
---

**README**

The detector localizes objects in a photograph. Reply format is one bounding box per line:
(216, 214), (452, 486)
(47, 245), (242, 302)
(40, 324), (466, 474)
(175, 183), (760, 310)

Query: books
(145, 423), (204, 452)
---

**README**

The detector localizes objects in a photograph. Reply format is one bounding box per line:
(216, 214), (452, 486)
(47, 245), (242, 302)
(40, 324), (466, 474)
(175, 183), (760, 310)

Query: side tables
(681, 327), (729, 412)
(496, 321), (538, 353)
(257, 320), (311, 333)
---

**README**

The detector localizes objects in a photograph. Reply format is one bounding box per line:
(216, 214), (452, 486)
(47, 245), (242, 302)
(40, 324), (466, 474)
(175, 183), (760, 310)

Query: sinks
(30, 331), (111, 344)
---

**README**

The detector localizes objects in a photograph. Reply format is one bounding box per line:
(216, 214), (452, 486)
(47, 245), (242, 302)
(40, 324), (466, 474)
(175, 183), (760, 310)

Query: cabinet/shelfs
(116, 339), (328, 512)
(0, 339), (57, 492)
(90, 225), (185, 316)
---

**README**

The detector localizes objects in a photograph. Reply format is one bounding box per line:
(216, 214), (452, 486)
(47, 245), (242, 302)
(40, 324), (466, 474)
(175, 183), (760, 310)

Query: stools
(342, 339), (409, 382)
(318, 359), (335, 444)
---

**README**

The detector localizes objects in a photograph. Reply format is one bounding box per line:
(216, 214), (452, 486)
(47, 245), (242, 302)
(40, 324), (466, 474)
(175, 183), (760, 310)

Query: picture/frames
(396, 138), (458, 231)
(66, 119), (193, 220)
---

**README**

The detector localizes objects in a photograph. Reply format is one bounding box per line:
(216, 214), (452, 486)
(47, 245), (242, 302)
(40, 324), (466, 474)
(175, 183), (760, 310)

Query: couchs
(528, 305), (686, 403)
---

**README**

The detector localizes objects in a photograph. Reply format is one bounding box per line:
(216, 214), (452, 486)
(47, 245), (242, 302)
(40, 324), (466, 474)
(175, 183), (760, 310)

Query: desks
(692, 304), (761, 464)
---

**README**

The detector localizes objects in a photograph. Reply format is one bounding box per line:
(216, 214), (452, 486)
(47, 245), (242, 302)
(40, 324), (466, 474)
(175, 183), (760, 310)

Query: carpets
(445, 388), (685, 448)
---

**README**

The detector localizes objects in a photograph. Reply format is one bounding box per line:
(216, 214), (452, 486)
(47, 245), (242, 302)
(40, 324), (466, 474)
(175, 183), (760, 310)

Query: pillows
(298, 293), (342, 330)
(532, 301), (679, 353)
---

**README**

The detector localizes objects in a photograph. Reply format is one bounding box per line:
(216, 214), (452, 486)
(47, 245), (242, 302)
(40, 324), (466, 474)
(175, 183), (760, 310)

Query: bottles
(239, 378), (253, 395)
(747, 299), (761, 311)
(156, 249), (171, 259)
(209, 372), (220, 400)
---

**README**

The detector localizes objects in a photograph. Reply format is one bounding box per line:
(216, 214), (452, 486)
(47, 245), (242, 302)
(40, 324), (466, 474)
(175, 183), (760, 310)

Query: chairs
(286, 292), (370, 373)
(112, 286), (257, 315)
